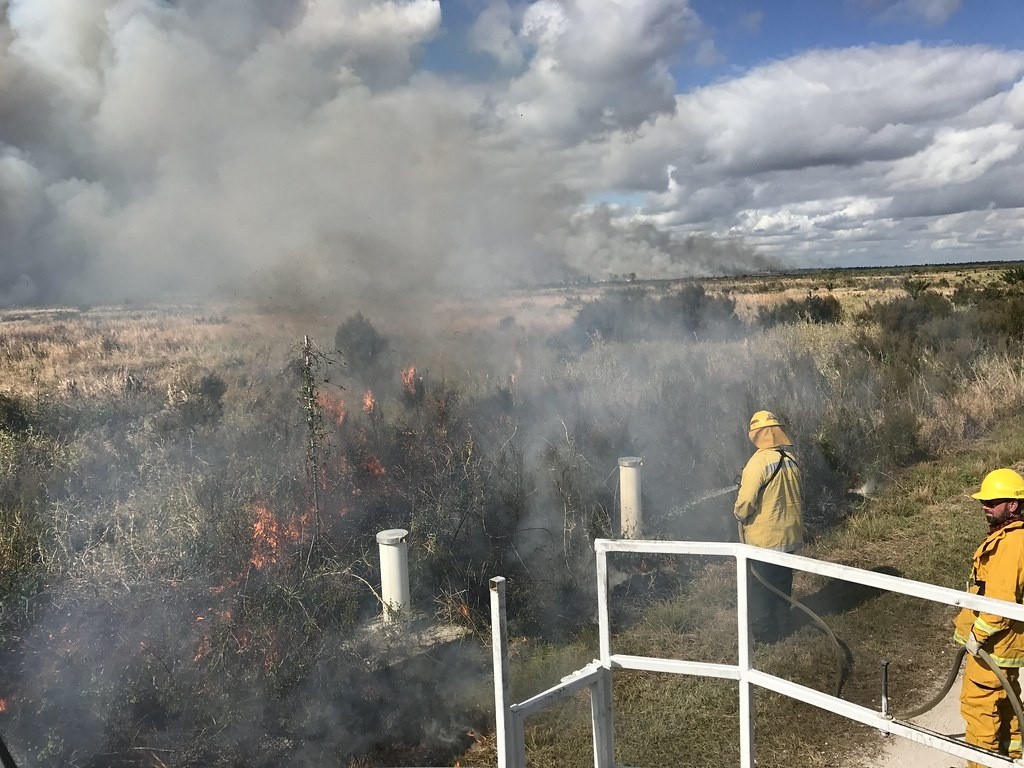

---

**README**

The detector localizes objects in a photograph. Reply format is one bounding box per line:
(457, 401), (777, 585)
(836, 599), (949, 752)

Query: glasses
(981, 499), (1005, 508)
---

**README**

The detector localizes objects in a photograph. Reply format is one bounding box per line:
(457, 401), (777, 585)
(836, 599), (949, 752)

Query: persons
(734, 411), (801, 644)
(949, 469), (1024, 768)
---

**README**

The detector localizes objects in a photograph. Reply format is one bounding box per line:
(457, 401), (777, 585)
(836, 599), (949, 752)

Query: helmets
(747, 411), (786, 432)
(971, 469), (1024, 499)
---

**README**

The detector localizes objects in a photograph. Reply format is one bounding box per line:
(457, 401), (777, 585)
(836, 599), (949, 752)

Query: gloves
(965, 632), (983, 658)
